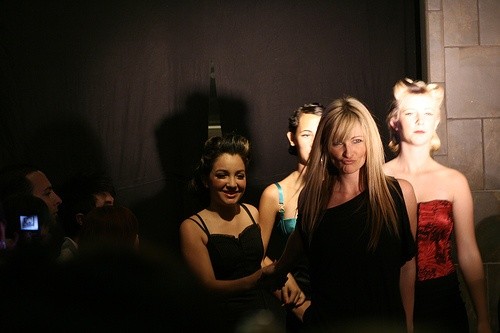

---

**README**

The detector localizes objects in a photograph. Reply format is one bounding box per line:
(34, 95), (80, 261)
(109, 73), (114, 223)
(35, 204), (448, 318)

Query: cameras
(19, 214), (39, 231)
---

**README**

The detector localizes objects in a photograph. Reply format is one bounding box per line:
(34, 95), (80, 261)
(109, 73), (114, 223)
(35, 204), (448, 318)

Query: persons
(268, 97), (417, 333)
(260, 102), (324, 332)
(382, 78), (489, 333)
(180, 135), (306, 333)
(0, 167), (142, 333)
